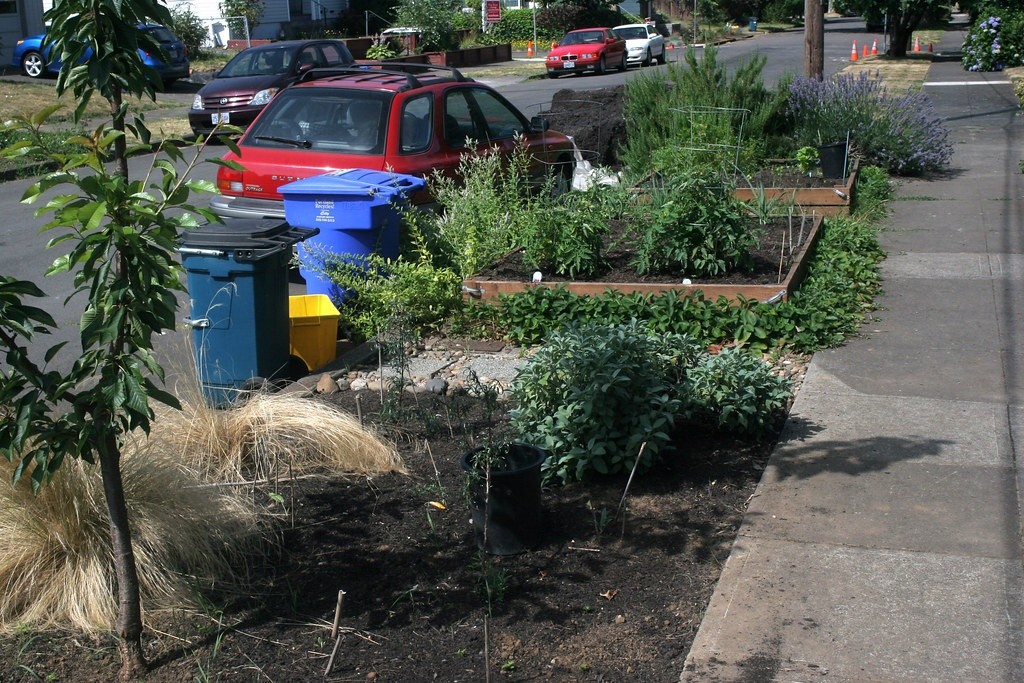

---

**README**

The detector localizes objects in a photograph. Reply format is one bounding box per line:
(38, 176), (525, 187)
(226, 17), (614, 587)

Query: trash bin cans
(277, 168), (429, 341)
(175, 217), (321, 410)
(748, 17), (758, 32)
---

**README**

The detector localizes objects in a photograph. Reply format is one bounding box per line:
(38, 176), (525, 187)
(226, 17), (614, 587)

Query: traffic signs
(485, 0), (502, 22)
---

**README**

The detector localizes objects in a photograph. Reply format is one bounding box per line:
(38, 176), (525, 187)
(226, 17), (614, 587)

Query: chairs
(347, 99), (383, 138)
(417, 112), (462, 145)
(640, 32), (647, 38)
(300, 52), (313, 63)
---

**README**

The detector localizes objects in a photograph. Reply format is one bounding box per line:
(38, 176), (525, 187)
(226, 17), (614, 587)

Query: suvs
(188, 40), (579, 225)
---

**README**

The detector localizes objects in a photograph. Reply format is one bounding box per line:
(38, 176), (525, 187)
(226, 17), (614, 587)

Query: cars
(10, 11), (189, 90)
(546, 28), (628, 79)
(612, 23), (666, 67)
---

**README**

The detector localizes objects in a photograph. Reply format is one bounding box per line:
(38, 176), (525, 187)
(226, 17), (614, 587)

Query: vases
(458, 441), (546, 557)
(817, 140), (846, 178)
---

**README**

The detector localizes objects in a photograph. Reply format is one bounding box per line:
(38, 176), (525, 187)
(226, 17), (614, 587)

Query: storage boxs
(289, 294), (341, 371)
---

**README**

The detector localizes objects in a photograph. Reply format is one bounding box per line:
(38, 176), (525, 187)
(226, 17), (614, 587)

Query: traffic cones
(914, 36), (921, 51)
(927, 43), (933, 52)
(666, 45), (675, 50)
(527, 40), (534, 57)
(862, 44), (869, 56)
(871, 39), (877, 55)
(850, 39), (858, 61)
